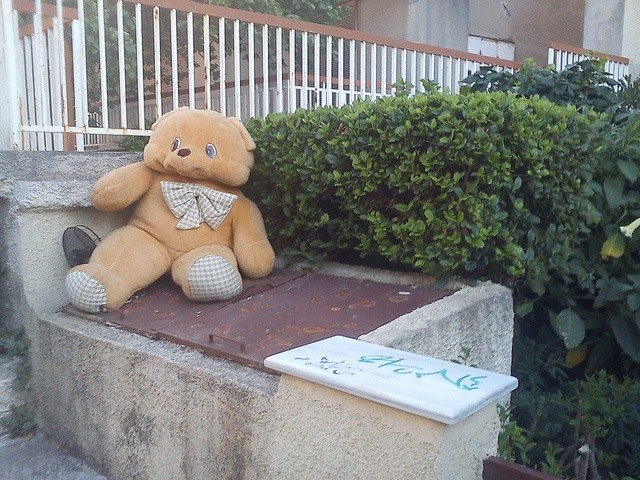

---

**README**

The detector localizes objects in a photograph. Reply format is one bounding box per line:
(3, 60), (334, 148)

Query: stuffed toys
(65, 106), (276, 314)
